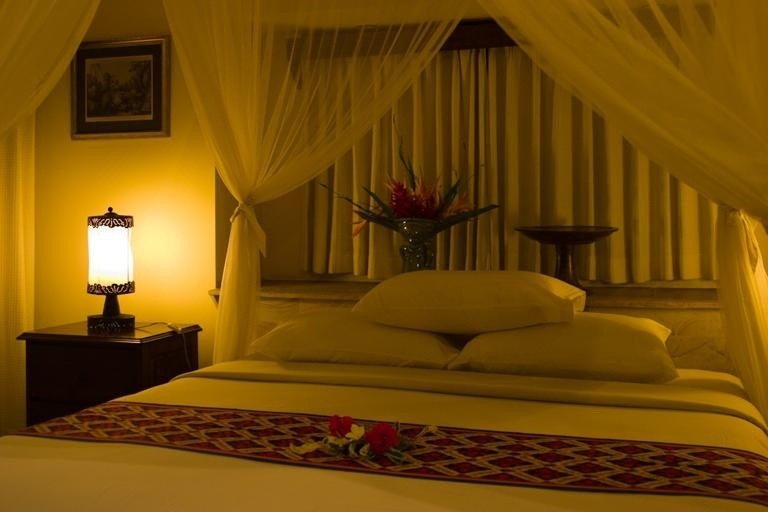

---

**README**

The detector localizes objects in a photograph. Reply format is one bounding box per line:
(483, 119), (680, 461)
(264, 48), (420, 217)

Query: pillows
(351, 270), (586, 333)
(248, 308), (459, 368)
(448, 312), (677, 382)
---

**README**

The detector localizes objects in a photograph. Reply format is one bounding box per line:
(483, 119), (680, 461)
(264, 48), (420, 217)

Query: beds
(0, 362), (768, 512)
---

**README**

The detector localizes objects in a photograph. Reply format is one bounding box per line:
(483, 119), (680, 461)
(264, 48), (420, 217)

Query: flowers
(321, 139), (501, 234)
(287, 414), (437, 473)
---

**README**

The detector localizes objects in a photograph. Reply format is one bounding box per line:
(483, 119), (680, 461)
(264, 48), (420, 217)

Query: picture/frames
(68, 32), (173, 141)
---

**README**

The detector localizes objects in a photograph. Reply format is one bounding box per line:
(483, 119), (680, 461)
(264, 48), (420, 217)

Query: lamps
(85, 207), (138, 332)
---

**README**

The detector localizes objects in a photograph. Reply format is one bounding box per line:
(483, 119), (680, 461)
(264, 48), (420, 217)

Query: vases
(394, 219), (435, 270)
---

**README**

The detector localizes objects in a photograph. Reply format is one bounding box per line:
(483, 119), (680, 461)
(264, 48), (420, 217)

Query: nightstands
(16, 318), (203, 429)
